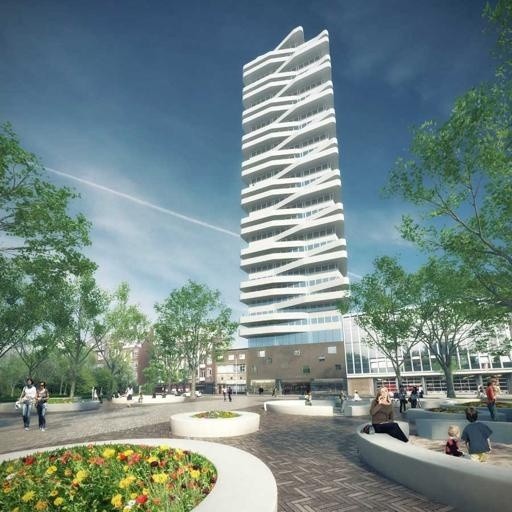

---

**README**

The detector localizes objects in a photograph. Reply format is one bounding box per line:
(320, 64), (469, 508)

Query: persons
(485, 382), (498, 421)
(125, 385), (131, 396)
(303, 389), (313, 406)
(351, 390), (362, 402)
(96, 387), (103, 404)
(338, 389), (347, 408)
(460, 406), (493, 464)
(93, 386), (98, 401)
(409, 386), (419, 409)
(33, 381), (50, 432)
(418, 384), (424, 398)
(221, 385), (227, 401)
(398, 385), (408, 413)
(271, 385), (278, 400)
(126, 386), (134, 409)
(368, 386), (409, 443)
(15, 377), (37, 433)
(257, 385), (264, 397)
(444, 425), (464, 458)
(226, 386), (233, 402)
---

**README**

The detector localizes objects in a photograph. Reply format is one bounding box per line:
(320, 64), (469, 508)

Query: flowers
(0, 444), (214, 510)
